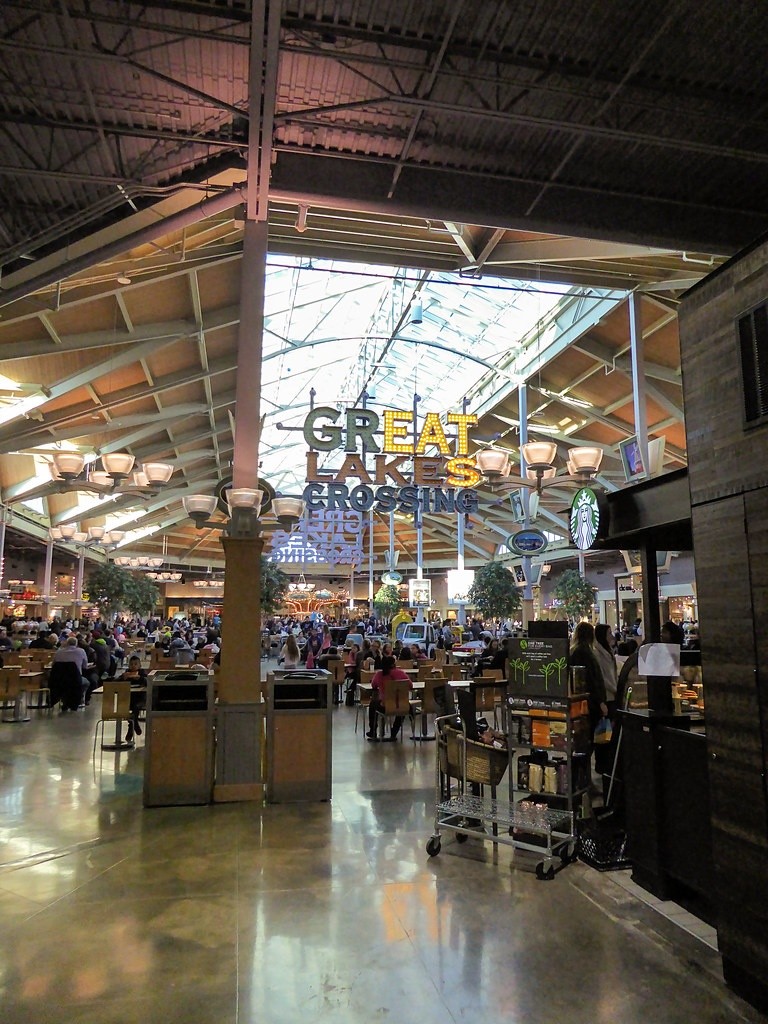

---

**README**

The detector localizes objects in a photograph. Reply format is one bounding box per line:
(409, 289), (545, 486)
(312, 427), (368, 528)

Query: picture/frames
(617, 435), (647, 482)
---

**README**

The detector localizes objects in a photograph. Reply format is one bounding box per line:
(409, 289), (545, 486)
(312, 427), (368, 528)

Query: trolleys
(426, 713), (580, 881)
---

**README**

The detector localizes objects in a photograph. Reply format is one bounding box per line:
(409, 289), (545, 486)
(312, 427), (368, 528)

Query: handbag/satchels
(594, 716), (612, 743)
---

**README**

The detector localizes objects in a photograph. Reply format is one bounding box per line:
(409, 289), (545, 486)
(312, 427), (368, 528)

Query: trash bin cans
(143, 669), (215, 808)
(267, 668), (332, 804)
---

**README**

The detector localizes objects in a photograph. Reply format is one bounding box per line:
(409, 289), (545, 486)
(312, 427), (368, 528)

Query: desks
(377, 668), (443, 680)
(356, 680), (508, 741)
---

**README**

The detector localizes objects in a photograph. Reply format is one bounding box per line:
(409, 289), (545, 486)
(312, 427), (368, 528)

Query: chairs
(1, 637), (509, 750)
(434, 714), (516, 820)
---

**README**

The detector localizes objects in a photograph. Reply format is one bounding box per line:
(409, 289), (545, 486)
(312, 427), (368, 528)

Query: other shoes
(391, 727), (398, 738)
(126, 728), (133, 742)
(134, 724), (142, 735)
(366, 728), (377, 738)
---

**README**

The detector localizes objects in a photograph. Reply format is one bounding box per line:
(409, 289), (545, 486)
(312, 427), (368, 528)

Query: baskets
(440, 730), (514, 786)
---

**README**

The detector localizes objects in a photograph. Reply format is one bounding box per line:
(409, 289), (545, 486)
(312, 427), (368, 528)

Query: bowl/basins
(443, 722), (466, 765)
(456, 734), (516, 785)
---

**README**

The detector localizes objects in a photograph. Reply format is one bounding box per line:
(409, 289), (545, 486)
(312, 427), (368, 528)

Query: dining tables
(91, 684), (149, 693)
(344, 663), (356, 667)
(19, 672), (44, 676)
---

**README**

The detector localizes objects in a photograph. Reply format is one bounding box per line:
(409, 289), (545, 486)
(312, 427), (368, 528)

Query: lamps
(541, 565), (551, 577)
(115, 556), (162, 571)
(316, 588), (334, 599)
(194, 580), (224, 588)
(182, 486), (308, 539)
(9, 579), (34, 588)
(146, 572), (182, 583)
(289, 570), (315, 593)
(476, 442), (603, 494)
(1, 451), (174, 549)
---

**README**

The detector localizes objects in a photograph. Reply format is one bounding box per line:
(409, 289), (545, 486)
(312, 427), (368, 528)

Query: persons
(568, 622), (609, 794)
(662, 621), (701, 683)
(0, 612), (642, 742)
(594, 625), (617, 774)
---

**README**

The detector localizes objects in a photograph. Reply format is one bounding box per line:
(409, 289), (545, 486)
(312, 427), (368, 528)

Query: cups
(370, 665), (374, 671)
